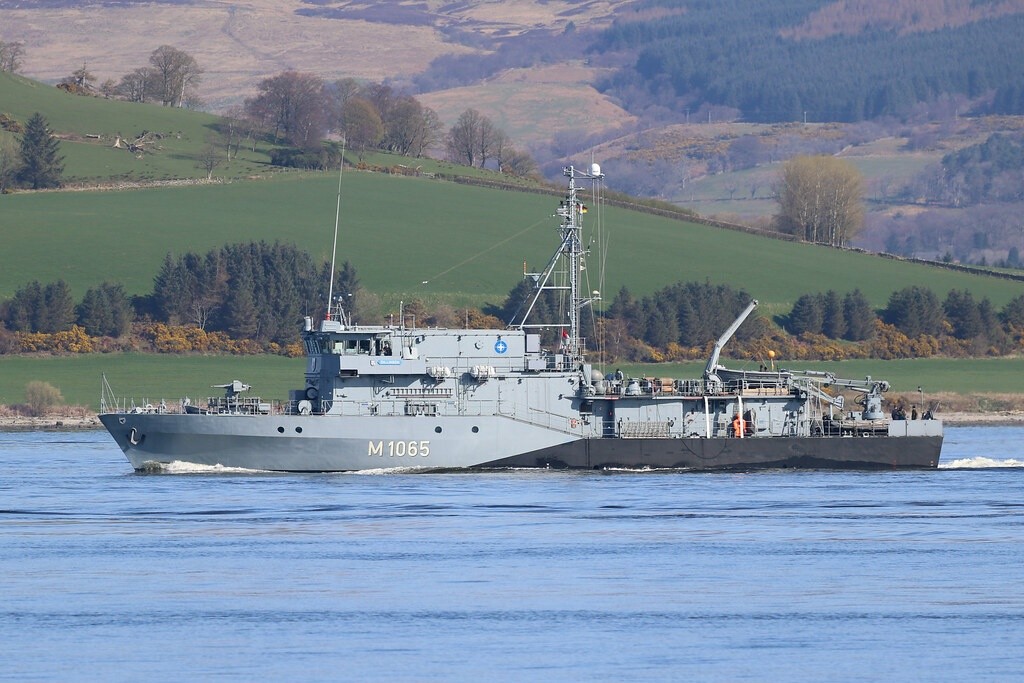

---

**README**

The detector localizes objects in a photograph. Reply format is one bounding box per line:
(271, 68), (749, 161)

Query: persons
(891, 405), (934, 421)
(614, 368), (623, 388)
(732, 413), (747, 438)
(823, 412), (841, 431)
(605, 372), (614, 386)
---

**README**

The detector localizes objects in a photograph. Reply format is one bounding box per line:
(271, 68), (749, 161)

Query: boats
(94, 138), (945, 480)
